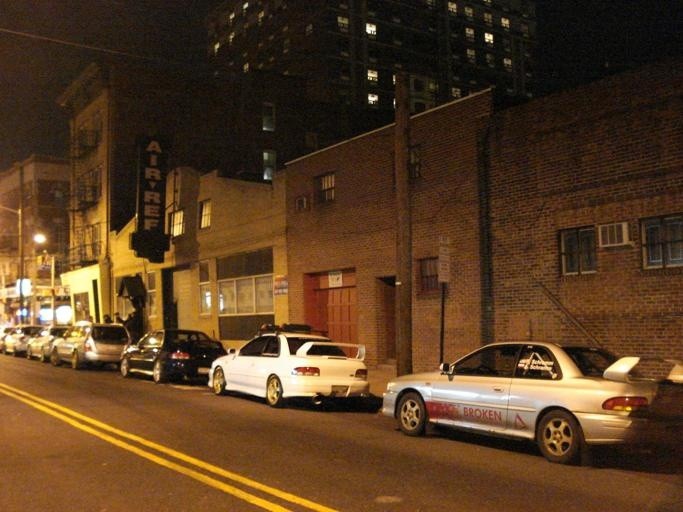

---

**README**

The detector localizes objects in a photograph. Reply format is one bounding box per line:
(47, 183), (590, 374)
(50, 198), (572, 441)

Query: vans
(48, 320), (131, 370)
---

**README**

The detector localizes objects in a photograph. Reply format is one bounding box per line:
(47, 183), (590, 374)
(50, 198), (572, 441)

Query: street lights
(29, 228), (46, 325)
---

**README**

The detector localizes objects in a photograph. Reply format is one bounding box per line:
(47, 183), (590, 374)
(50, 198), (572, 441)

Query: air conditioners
(597, 221), (633, 249)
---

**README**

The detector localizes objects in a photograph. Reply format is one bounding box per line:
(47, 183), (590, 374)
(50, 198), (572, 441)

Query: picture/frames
(199, 281), (211, 315)
(253, 273), (275, 316)
(234, 275), (256, 316)
(216, 278), (236, 317)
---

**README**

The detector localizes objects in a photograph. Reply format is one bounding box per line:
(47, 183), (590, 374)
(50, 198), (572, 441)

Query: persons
(82, 311), (139, 342)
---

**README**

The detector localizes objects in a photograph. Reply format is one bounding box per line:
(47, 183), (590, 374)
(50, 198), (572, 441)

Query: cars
(382, 341), (682, 463)
(25, 325), (73, 361)
(206, 324), (369, 408)
(119, 328), (227, 384)
(0, 324), (46, 356)
(0, 324), (15, 339)
